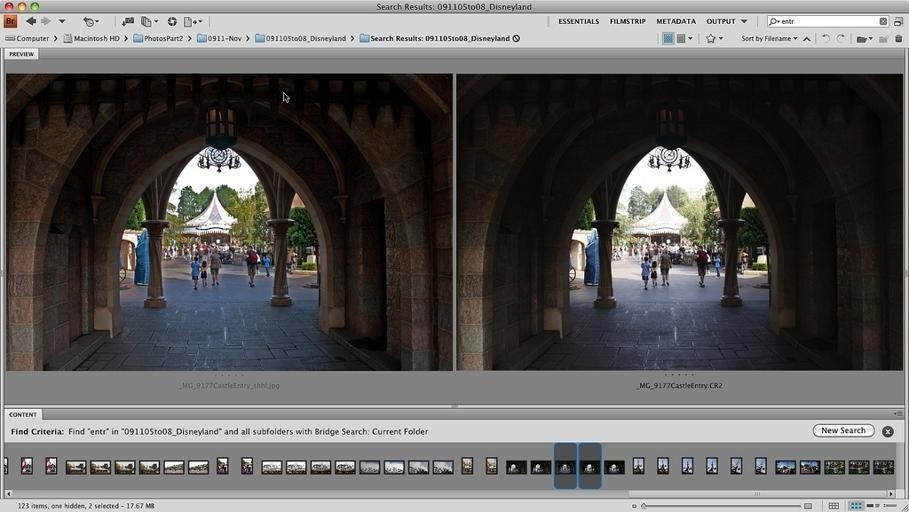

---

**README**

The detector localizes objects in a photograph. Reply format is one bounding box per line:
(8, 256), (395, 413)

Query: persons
(630, 237), (749, 288)
(162, 238), (298, 289)
